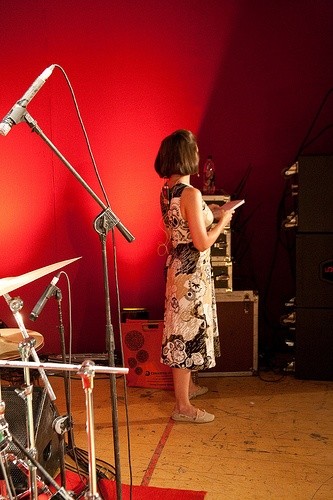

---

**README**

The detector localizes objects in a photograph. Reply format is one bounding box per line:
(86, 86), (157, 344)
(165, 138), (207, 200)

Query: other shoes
(187, 386), (208, 400)
(171, 407), (215, 423)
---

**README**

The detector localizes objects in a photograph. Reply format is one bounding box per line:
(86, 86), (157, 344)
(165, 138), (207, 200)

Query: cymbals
(0, 256), (83, 300)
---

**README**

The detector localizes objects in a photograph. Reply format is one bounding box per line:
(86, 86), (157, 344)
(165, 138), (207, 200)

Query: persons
(154, 130), (235, 422)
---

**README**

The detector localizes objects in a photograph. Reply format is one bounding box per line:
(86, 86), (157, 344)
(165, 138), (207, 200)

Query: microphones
(29, 272), (61, 322)
(0, 64), (55, 136)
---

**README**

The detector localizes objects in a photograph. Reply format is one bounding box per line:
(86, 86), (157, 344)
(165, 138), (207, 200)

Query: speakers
(294, 308), (333, 381)
(296, 153), (333, 234)
(290, 233), (333, 308)
(0, 387), (63, 500)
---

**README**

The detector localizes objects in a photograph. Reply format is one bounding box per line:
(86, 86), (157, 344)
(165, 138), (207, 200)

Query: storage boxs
(203, 196), (232, 292)
(197, 290), (259, 376)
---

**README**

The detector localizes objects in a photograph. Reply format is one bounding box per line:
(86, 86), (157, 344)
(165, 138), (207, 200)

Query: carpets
(38, 469), (206, 500)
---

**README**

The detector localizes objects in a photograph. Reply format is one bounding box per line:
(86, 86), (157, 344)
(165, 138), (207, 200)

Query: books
(213, 199), (244, 217)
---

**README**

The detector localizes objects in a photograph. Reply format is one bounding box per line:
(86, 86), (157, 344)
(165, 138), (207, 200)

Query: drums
(0, 328), (45, 388)
